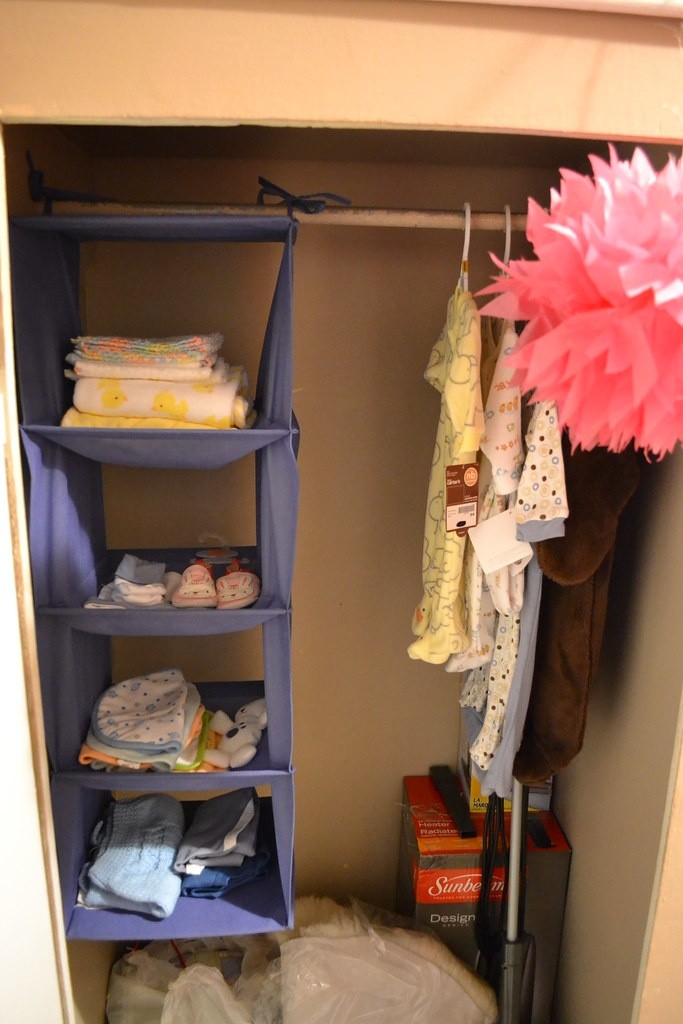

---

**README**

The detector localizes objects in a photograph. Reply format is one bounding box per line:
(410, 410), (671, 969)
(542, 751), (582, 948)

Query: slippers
(215, 571), (260, 609)
(171, 565), (217, 607)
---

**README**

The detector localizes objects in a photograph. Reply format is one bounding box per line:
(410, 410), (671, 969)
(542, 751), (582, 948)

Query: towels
(60, 323), (259, 431)
(75, 791), (182, 921)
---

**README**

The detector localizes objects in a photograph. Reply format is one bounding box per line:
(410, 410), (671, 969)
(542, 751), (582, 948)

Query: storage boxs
(393, 775), (485, 971)
(469, 809), (572, 1024)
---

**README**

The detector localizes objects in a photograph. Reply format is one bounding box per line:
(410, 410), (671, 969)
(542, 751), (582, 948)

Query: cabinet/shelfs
(501, 202), (515, 307)
(14, 188), (313, 943)
(455, 200), (474, 296)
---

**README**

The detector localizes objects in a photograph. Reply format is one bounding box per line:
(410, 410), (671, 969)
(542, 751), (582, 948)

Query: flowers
(461, 141), (683, 466)
